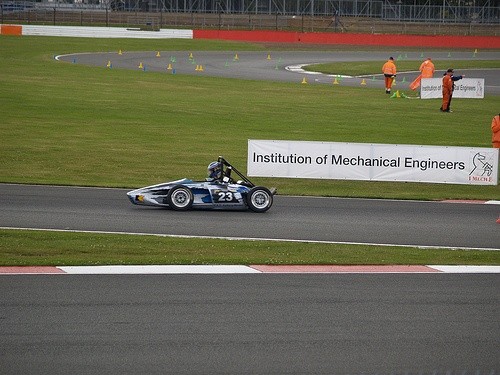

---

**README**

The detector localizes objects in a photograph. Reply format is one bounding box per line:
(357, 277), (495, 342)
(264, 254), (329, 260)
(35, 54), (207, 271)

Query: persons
(207, 161), (221, 182)
(439, 69), (465, 112)
(382, 56), (397, 94)
(491, 114), (500, 148)
(420, 57), (435, 78)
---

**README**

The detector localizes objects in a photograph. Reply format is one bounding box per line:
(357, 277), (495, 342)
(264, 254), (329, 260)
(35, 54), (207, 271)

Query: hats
(447, 70), (454, 73)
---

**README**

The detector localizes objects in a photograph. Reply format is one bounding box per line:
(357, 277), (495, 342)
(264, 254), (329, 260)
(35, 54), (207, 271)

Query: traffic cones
(361, 79), (366, 85)
(170, 53), (196, 64)
(156, 51), (160, 56)
(391, 90), (400, 98)
(333, 74), (342, 84)
(107, 61), (111, 66)
(195, 65), (203, 71)
(232, 53), (238, 62)
(301, 77), (307, 84)
(138, 62), (143, 68)
(402, 77), (406, 82)
(168, 64), (172, 69)
(118, 50), (122, 55)
(397, 49), (478, 60)
(267, 54), (271, 60)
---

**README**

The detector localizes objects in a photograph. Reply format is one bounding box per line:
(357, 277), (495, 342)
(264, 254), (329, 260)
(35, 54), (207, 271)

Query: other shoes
(386, 90), (391, 94)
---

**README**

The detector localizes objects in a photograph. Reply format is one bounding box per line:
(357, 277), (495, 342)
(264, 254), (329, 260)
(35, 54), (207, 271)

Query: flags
(409, 74), (422, 91)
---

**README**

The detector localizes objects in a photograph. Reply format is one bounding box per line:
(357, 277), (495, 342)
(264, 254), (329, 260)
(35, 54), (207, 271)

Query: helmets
(208, 161), (221, 170)
